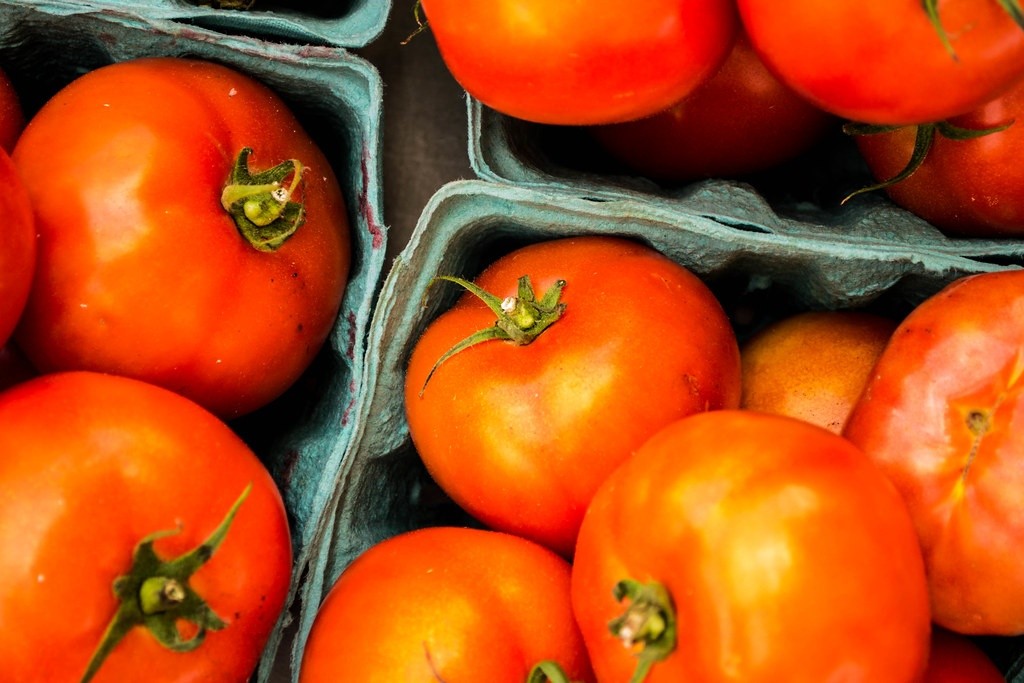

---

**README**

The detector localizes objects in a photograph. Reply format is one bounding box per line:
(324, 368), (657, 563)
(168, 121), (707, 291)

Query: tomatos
(0, 1), (1024, 682)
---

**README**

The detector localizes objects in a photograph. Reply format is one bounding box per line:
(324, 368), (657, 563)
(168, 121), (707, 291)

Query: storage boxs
(0, 0), (1024, 683)
